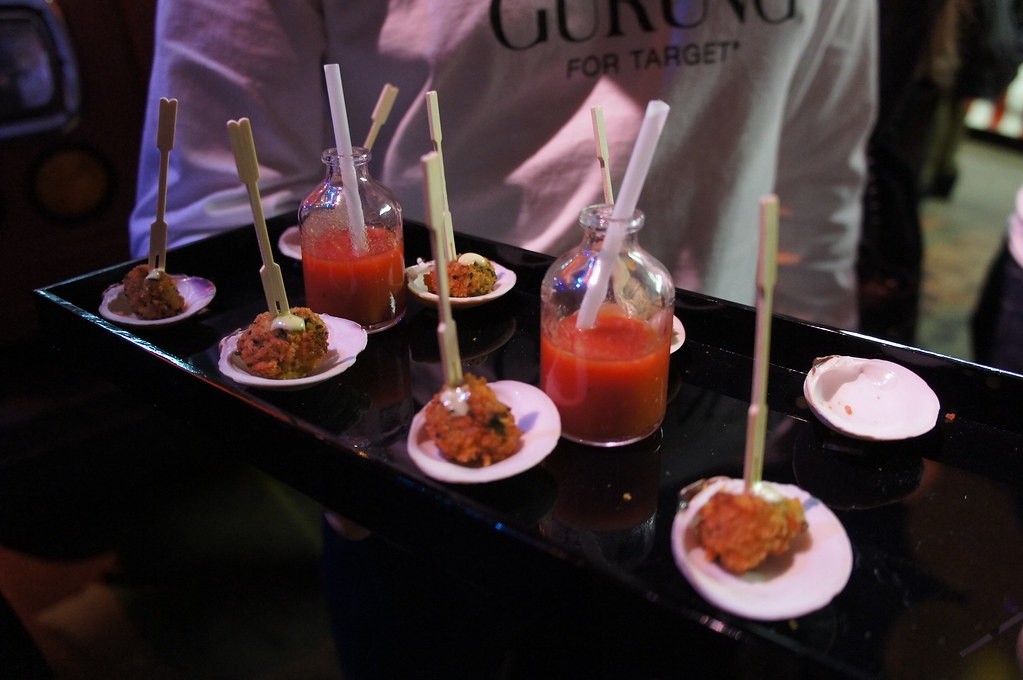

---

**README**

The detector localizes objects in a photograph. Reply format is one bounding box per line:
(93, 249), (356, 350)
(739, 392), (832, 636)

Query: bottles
(296, 148), (404, 337)
(538, 205), (676, 448)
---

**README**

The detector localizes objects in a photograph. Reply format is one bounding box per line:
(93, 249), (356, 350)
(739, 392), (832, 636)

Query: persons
(854, 2), (1023, 376)
(130, 0), (880, 679)
